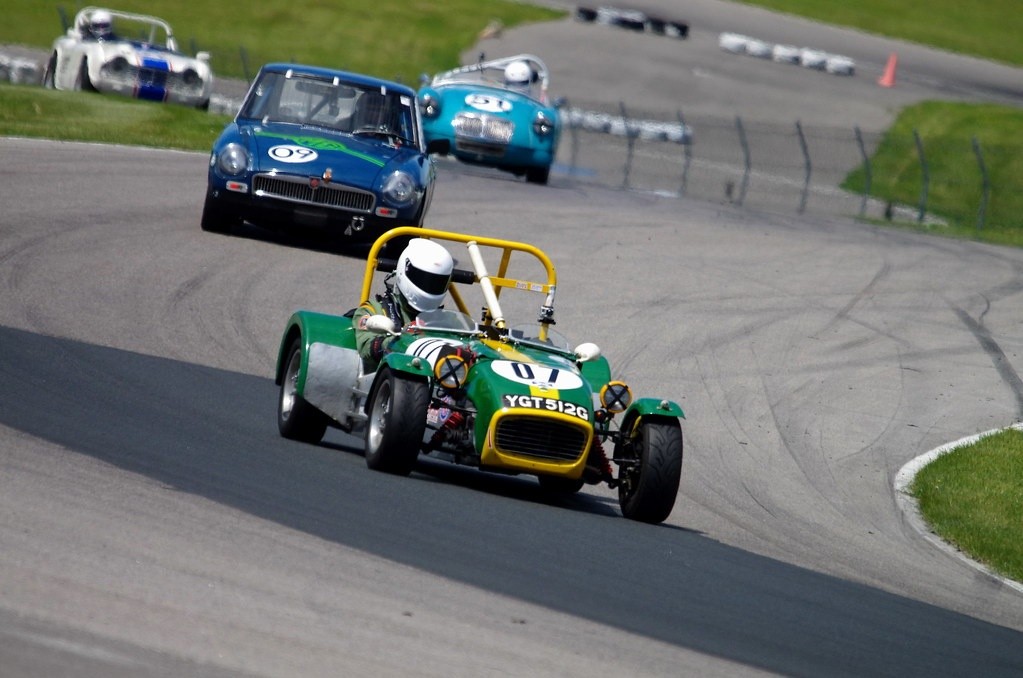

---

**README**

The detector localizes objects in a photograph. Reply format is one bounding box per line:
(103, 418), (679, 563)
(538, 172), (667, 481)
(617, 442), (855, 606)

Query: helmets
(396, 238), (453, 312)
(505, 61), (532, 93)
(90, 11), (111, 38)
(356, 91), (391, 111)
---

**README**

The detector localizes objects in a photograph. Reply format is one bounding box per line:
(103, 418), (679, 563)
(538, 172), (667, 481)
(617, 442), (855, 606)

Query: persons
(503, 62), (548, 97)
(333, 90), (403, 146)
(82, 9), (121, 43)
(351, 237), (464, 380)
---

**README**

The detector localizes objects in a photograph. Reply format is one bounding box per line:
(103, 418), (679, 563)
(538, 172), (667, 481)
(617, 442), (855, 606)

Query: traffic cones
(879, 51), (898, 89)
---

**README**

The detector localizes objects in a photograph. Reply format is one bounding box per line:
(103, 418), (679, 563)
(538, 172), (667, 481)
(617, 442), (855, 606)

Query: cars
(413, 53), (561, 186)
(199, 62), (452, 267)
(40, 7), (217, 115)
